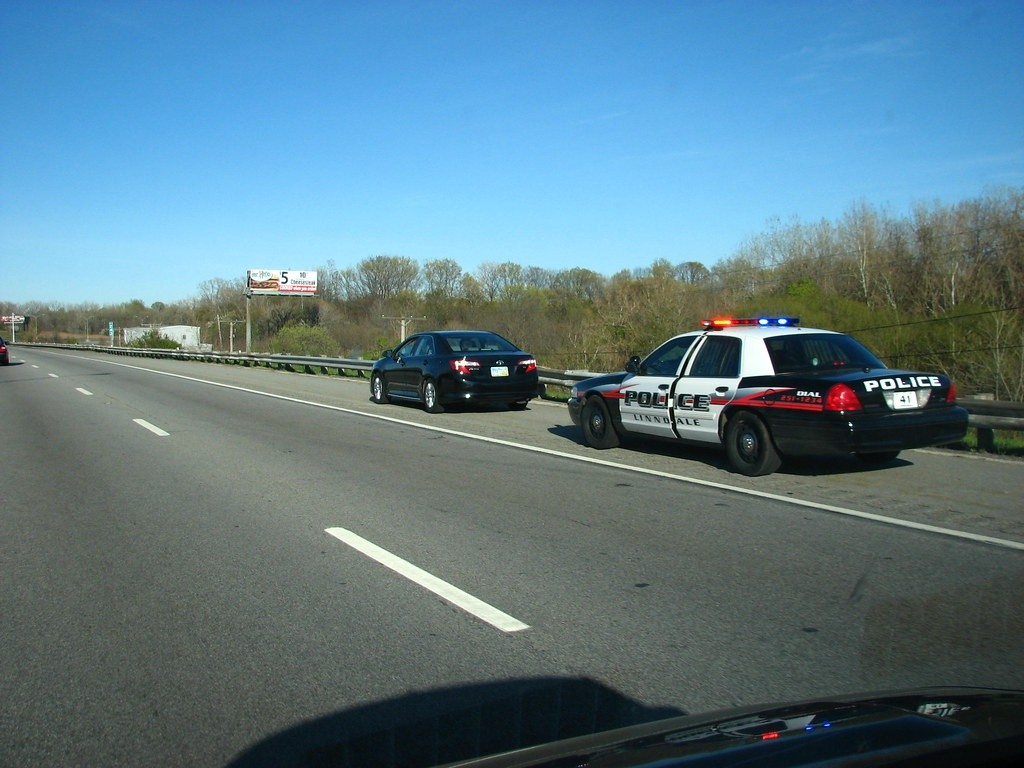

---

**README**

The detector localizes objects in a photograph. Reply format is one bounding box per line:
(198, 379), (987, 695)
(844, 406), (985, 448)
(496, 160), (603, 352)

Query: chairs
(460, 339), (476, 351)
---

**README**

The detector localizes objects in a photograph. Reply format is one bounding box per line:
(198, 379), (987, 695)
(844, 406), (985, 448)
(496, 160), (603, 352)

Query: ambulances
(567, 316), (969, 477)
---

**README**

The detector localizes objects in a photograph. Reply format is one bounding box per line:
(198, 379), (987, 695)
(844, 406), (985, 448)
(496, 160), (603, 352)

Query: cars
(370, 330), (539, 414)
(0, 336), (9, 366)
